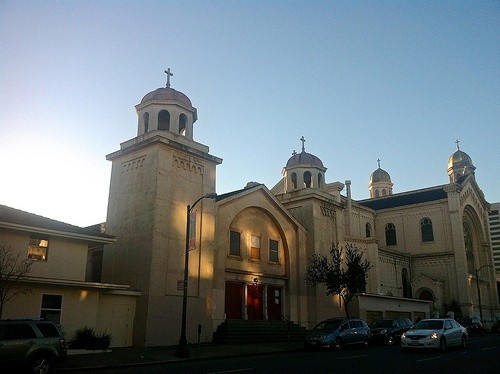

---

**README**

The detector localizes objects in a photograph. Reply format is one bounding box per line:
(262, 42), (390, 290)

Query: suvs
(0, 318), (67, 374)
(368, 317), (415, 347)
(305, 316), (370, 352)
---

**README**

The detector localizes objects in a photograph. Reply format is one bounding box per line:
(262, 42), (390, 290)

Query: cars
(401, 319), (468, 353)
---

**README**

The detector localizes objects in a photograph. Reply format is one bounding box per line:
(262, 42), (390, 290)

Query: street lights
(177, 192), (217, 358)
(475, 265), (494, 329)
